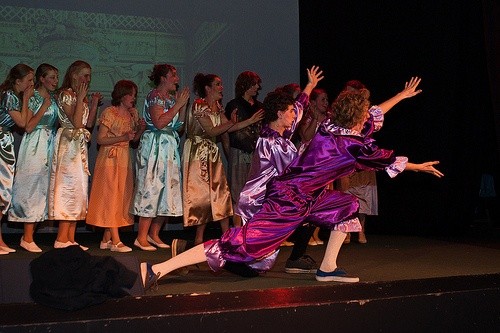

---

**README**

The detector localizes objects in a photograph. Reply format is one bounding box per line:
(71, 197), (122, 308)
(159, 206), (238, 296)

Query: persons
(225, 70), (264, 227)
(181, 72), (264, 244)
(140, 76), (443, 291)
(8, 63), (59, 252)
(85, 80), (146, 253)
(337, 81), (378, 244)
(171, 65), (324, 278)
(275, 83), (333, 246)
(48, 60), (103, 251)
(0, 64), (35, 255)
(129, 64), (190, 251)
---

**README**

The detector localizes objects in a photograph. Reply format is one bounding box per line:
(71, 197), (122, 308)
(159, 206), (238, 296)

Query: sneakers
(140, 262), (160, 290)
(172, 238), (192, 274)
(285, 255), (319, 274)
(316, 267), (359, 282)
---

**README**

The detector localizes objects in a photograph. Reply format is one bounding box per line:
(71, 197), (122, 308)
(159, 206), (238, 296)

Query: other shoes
(344, 233), (350, 243)
(284, 242), (294, 246)
(308, 241), (317, 245)
(359, 233), (367, 244)
(316, 241), (323, 244)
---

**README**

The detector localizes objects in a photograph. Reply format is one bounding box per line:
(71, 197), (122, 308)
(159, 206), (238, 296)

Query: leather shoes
(20, 239), (42, 252)
(134, 239), (156, 250)
(147, 235), (170, 247)
(100, 241), (112, 249)
(54, 241), (73, 248)
(72, 241), (89, 250)
(110, 242), (132, 252)
(0, 250), (9, 254)
(0, 246), (16, 252)
